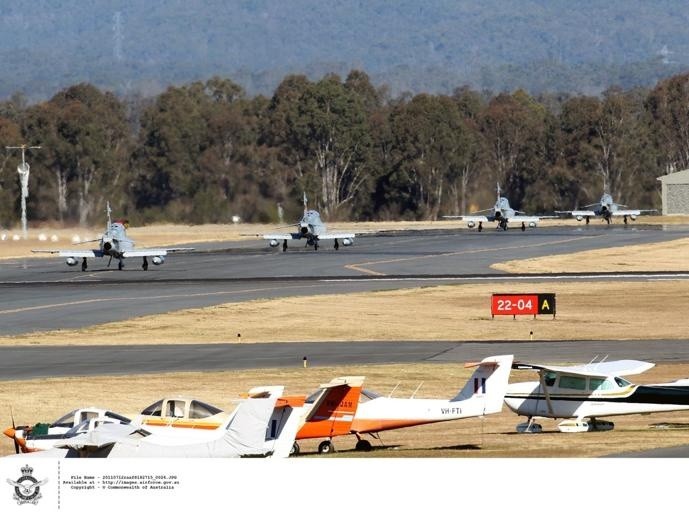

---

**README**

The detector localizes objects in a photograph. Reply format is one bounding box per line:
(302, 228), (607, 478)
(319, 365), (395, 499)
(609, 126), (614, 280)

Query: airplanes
(31, 201), (196, 272)
(442, 181), (658, 232)
(238, 192), (408, 252)
